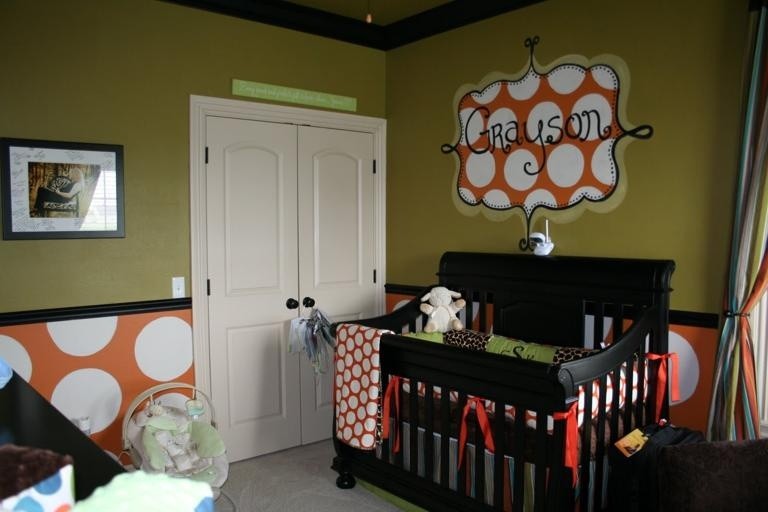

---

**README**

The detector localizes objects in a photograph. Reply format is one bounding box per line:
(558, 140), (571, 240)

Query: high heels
(30, 209), (40, 217)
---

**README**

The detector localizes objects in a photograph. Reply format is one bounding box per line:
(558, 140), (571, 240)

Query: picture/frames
(0, 137), (125, 240)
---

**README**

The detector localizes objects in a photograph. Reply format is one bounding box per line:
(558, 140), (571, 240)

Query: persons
(30, 167), (85, 218)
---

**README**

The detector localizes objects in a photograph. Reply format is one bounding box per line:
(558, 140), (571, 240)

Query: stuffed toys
(419, 286), (467, 333)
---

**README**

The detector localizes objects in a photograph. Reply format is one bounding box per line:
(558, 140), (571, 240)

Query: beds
(330, 251), (675, 512)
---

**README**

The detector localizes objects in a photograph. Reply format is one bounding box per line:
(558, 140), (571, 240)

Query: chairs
(122, 382), (237, 512)
(42, 176), (79, 217)
